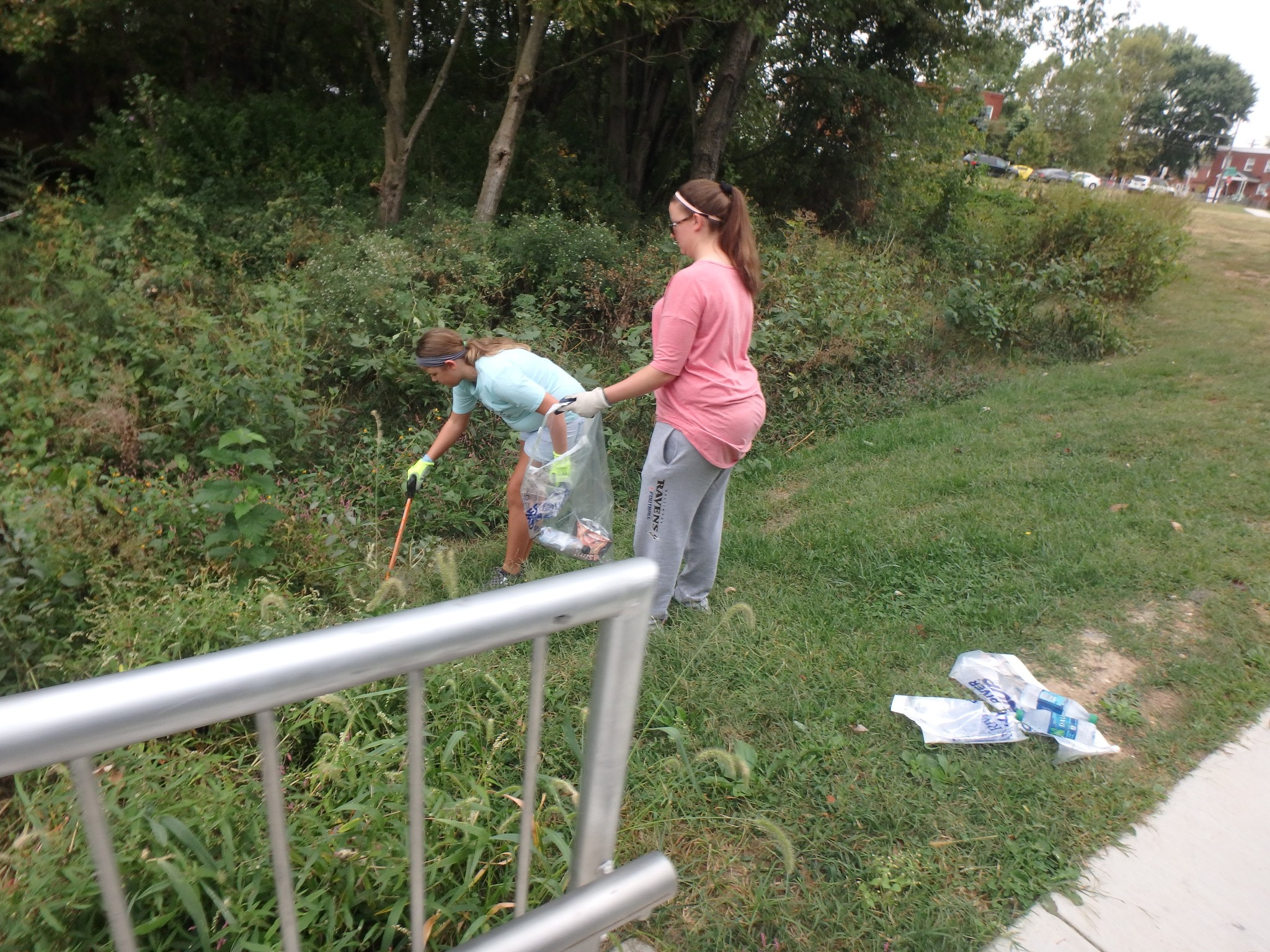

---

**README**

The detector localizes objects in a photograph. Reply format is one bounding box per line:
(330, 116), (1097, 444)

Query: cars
(1005, 164), (1034, 181)
(1028, 168), (1072, 184)
(1070, 171), (1101, 190)
(963, 152), (1019, 180)
(1127, 175), (1175, 198)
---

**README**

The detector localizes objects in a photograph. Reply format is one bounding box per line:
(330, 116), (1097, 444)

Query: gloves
(408, 455), (435, 491)
(549, 451), (571, 487)
(554, 387), (612, 419)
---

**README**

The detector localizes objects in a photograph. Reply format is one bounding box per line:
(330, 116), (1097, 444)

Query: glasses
(668, 214), (708, 234)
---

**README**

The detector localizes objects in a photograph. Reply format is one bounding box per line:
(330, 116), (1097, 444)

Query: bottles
(1015, 707), (1096, 746)
(1019, 683), (1098, 725)
(538, 526), (590, 556)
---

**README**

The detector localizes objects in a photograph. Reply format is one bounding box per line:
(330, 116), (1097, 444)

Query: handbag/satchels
(519, 401), (614, 567)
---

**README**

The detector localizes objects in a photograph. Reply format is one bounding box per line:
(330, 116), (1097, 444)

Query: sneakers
(649, 604), (712, 631)
(480, 561), (528, 591)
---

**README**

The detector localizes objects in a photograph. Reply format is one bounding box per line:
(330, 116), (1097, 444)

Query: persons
(557, 178), (767, 632)
(404, 328), (600, 593)
